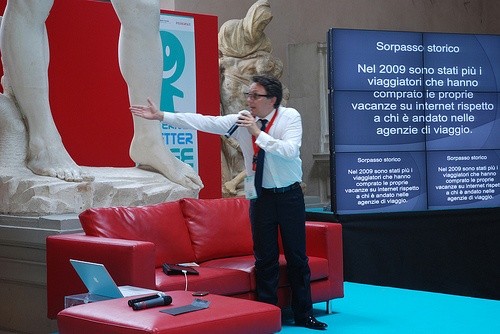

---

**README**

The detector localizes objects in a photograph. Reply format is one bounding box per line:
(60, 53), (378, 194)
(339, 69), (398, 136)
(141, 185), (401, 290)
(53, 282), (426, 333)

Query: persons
(129, 73), (329, 329)
(217, 0), (308, 198)
(0, 0), (205, 191)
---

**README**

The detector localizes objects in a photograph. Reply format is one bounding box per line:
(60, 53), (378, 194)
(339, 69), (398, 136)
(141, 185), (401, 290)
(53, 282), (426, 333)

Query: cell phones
(192, 291), (209, 295)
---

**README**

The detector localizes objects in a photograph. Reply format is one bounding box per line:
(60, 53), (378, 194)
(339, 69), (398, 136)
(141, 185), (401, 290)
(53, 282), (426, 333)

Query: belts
(262, 182), (300, 192)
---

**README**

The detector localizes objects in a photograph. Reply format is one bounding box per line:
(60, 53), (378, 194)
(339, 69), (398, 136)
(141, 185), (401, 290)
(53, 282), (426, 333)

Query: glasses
(243, 93), (272, 100)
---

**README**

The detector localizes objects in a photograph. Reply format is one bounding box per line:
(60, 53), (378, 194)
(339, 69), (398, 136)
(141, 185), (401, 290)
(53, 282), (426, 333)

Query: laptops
(70, 258), (159, 299)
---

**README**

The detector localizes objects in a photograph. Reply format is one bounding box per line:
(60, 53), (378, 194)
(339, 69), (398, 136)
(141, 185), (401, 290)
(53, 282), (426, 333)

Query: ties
(255, 120), (268, 193)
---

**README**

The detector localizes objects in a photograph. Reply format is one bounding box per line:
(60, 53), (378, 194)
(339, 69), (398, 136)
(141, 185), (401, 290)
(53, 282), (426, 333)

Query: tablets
(163, 262), (198, 275)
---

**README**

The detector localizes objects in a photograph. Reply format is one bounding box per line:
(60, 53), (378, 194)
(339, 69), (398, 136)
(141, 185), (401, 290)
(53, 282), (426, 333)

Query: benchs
(57, 290), (281, 334)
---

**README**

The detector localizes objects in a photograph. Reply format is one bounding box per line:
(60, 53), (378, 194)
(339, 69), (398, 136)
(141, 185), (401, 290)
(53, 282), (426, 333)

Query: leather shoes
(295, 316), (328, 329)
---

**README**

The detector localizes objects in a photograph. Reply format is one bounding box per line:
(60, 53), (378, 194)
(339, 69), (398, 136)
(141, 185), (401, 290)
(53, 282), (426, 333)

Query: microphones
(132, 296), (172, 310)
(225, 110), (250, 138)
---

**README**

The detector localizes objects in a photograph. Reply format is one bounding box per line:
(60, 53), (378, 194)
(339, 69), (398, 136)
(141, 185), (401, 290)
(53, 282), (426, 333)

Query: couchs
(46, 195), (345, 321)
